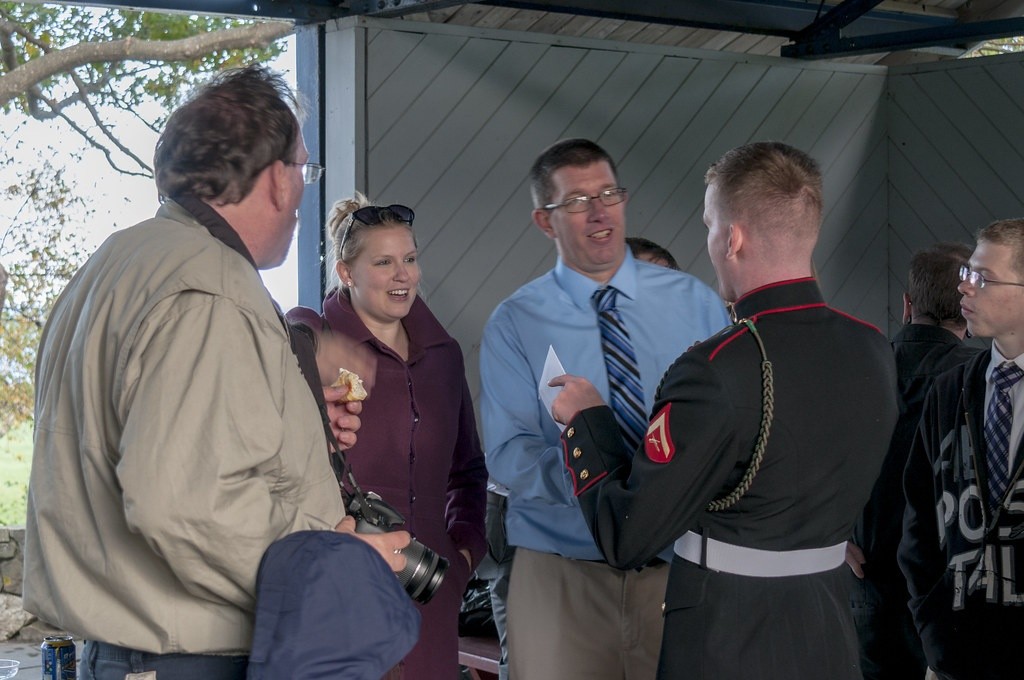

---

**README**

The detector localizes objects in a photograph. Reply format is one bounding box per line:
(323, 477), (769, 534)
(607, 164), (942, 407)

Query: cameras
(341, 490), (451, 606)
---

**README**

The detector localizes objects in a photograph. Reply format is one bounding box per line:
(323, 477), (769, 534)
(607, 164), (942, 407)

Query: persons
(896, 217), (1024, 680)
(852, 238), (982, 680)
(548, 140), (895, 680)
(478, 135), (734, 680)
(19, 64), (413, 680)
(626, 235), (679, 271)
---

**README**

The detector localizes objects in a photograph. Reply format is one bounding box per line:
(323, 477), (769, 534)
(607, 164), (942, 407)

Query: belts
(672, 530), (848, 577)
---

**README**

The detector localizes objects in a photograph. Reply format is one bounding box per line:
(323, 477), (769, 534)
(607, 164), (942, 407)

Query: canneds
(41, 634), (77, 680)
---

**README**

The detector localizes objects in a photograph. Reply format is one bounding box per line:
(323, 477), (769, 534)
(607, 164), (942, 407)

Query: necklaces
(284, 188), (487, 680)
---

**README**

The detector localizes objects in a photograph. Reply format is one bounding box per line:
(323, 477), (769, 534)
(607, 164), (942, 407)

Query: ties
(591, 285), (647, 456)
(983, 364), (1024, 517)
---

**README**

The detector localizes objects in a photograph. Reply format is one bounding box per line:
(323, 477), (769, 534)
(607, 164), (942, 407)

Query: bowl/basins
(0, 659), (20, 680)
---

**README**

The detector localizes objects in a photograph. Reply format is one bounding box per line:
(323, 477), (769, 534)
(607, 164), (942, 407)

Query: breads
(330, 368), (367, 401)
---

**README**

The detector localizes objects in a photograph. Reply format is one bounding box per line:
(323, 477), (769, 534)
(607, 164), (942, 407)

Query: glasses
(541, 188), (627, 213)
(958, 265), (1024, 289)
(278, 159), (326, 184)
(339, 205), (416, 261)
